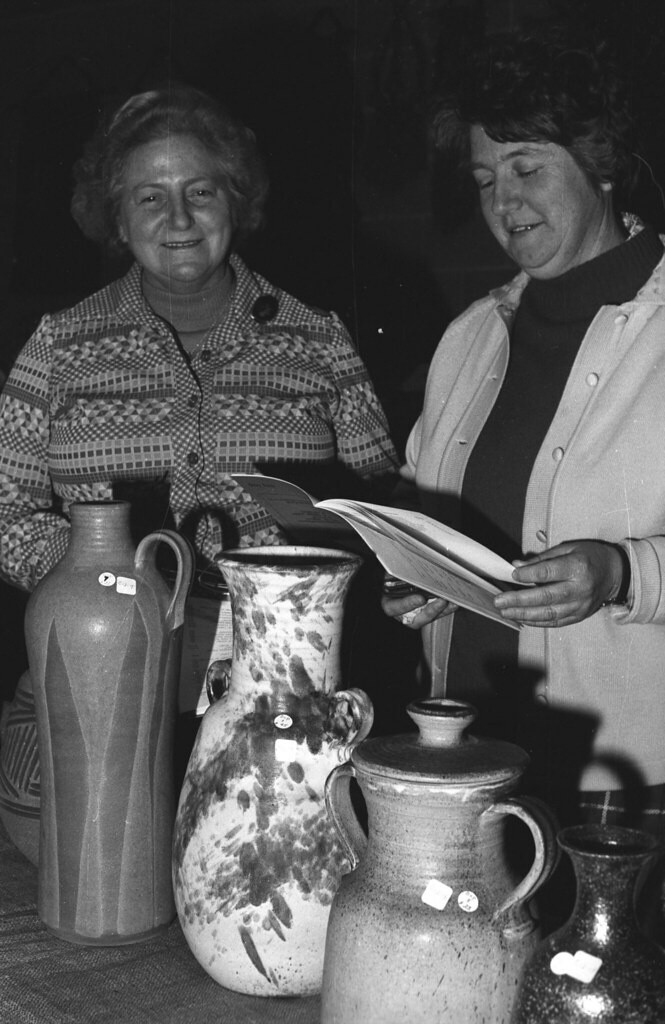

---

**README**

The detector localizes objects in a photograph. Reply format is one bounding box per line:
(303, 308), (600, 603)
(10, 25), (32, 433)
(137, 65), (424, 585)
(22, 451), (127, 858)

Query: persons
(381, 20), (665, 845)
(0, 84), (404, 592)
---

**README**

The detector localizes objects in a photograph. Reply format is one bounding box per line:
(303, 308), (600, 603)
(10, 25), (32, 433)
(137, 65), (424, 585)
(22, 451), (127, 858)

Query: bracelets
(607, 540), (630, 610)
